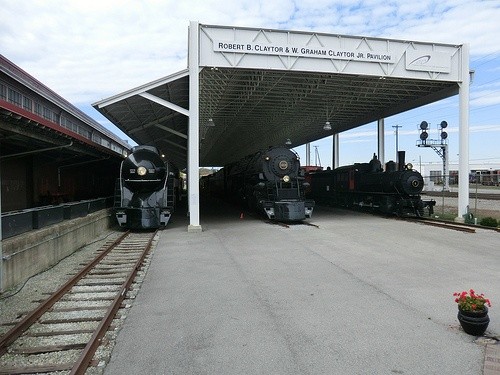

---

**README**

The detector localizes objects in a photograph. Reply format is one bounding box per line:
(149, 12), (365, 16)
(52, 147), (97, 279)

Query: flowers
(453, 289), (491, 311)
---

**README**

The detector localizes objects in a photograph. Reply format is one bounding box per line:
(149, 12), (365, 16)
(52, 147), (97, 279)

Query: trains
(300, 153), (436, 218)
(199, 145), (316, 222)
(115, 143), (184, 230)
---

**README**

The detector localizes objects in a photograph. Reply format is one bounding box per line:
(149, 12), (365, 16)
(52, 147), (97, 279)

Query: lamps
(206, 118), (216, 127)
(285, 138), (292, 144)
(323, 121), (333, 130)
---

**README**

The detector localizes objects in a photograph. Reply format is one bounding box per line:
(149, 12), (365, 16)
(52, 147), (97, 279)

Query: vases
(453, 305), (491, 336)
